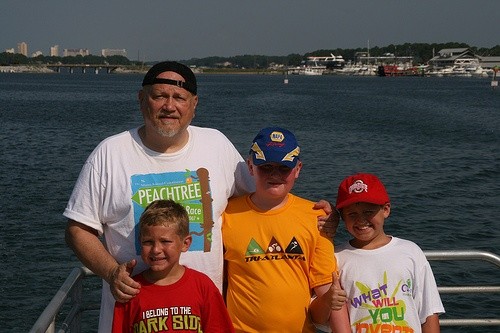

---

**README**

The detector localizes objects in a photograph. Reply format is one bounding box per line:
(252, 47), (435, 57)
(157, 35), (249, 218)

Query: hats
(336, 173), (390, 209)
(249, 127), (300, 168)
(142, 61), (196, 95)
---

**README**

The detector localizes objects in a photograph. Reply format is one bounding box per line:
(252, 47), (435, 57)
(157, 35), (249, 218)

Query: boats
(287, 52), (500, 78)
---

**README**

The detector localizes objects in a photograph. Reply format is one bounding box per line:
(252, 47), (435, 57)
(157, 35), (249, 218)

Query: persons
(111, 199), (234, 333)
(220, 127), (352, 332)
(63, 61), (340, 332)
(308, 174), (445, 333)
